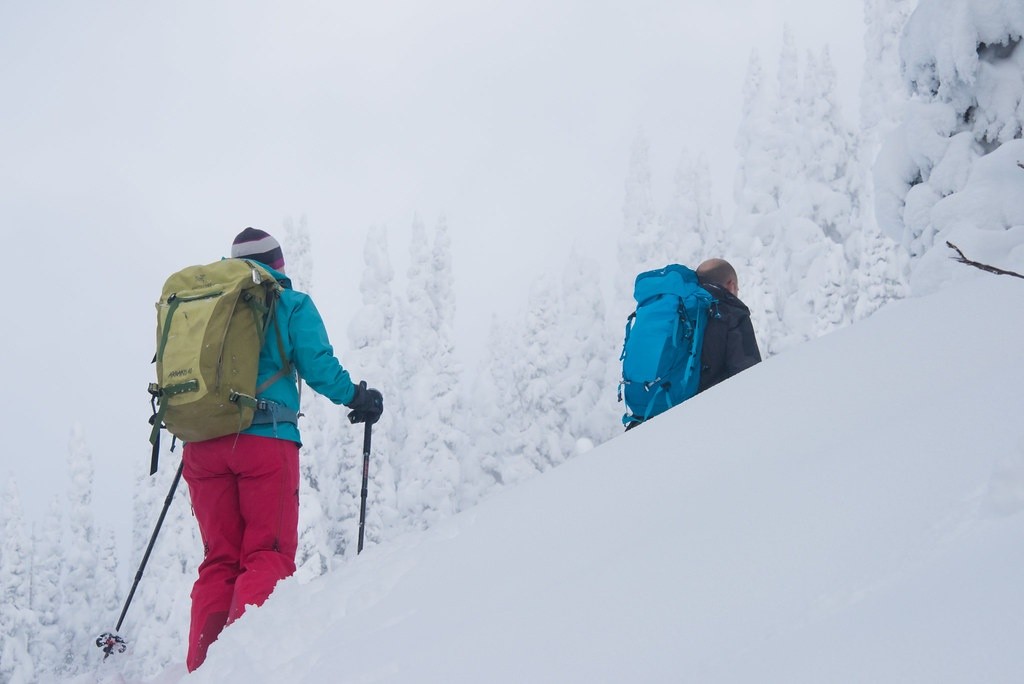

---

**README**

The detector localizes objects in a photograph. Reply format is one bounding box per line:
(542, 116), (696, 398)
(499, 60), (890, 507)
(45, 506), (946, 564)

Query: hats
(231, 226), (285, 269)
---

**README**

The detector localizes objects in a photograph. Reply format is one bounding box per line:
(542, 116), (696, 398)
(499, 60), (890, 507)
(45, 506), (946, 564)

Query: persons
(696, 257), (762, 394)
(180, 226), (383, 673)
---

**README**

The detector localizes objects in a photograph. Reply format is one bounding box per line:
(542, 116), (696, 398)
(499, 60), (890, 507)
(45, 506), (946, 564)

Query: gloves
(347, 380), (384, 426)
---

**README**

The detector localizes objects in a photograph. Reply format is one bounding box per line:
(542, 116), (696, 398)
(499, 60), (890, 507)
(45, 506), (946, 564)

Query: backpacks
(148, 257), (288, 476)
(617, 263), (721, 432)
